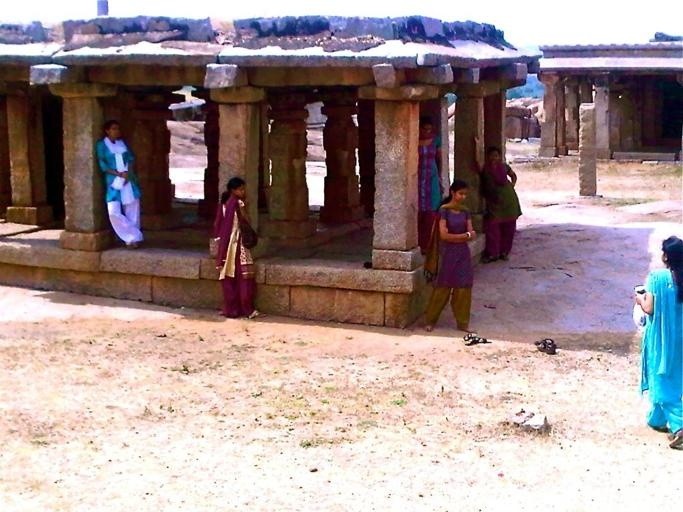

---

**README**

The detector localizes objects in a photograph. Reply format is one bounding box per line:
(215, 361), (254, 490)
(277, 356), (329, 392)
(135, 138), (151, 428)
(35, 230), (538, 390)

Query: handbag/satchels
(233, 197), (257, 249)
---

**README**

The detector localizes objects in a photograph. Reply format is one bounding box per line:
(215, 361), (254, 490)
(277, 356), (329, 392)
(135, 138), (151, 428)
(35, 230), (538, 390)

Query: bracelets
(118, 172), (122, 177)
(466, 230), (472, 239)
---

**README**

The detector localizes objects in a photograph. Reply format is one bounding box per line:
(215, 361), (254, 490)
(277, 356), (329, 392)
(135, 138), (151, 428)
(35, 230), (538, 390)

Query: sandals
(462, 333), (491, 346)
(534, 338), (556, 354)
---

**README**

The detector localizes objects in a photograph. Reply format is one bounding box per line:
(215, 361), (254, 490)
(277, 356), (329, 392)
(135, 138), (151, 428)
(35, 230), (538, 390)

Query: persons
(631, 233), (681, 451)
(414, 112), (446, 256)
(472, 134), (522, 265)
(94, 117), (146, 250)
(420, 179), (480, 333)
(211, 175), (266, 321)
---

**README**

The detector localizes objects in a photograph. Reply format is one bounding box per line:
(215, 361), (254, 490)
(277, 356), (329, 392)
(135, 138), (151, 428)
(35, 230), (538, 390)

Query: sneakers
(247, 310), (267, 320)
(480, 254), (508, 263)
(666, 431), (683, 447)
